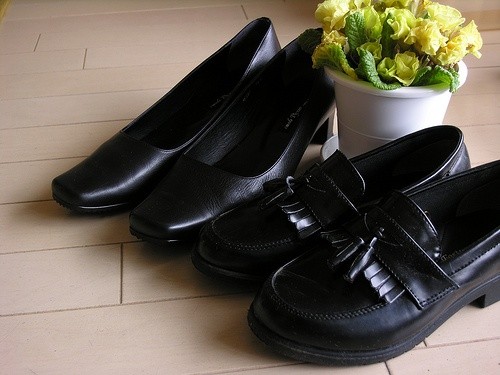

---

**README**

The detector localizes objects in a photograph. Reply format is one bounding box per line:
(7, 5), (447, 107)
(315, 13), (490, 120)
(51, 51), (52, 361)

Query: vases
(323, 60), (468, 158)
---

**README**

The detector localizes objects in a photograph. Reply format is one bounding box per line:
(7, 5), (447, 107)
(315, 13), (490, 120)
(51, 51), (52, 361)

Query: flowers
(310, 0), (483, 94)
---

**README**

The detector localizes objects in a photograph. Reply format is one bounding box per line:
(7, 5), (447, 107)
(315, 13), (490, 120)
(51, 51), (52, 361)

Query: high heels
(246, 160), (499, 366)
(189, 126), (473, 293)
(53, 14), (284, 214)
(128, 27), (335, 242)
